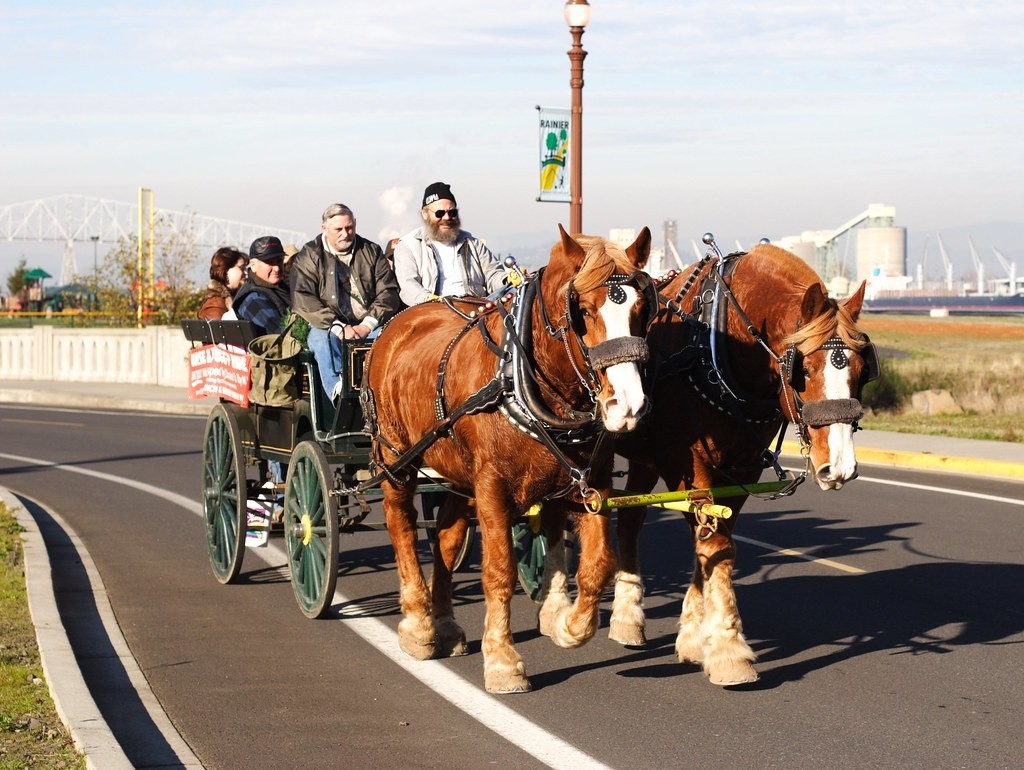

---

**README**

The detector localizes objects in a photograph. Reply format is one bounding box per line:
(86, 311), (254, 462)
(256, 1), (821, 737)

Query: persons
(290, 203), (400, 411)
(393, 181), (508, 308)
(195, 236), (403, 345)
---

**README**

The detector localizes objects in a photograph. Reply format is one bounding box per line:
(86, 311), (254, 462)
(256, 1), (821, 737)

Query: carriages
(179, 225), (879, 696)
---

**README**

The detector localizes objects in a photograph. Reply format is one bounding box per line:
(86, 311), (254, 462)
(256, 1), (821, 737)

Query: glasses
(429, 208), (458, 218)
(233, 265), (246, 270)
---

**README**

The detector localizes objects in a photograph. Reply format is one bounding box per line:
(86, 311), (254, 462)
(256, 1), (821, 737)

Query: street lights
(565, 0), (591, 234)
(91, 236), (100, 301)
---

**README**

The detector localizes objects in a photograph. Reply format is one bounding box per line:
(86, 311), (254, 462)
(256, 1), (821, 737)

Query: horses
(358, 222), (878, 695)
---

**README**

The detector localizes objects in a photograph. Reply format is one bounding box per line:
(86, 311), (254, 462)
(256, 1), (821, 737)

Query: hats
(249, 236), (289, 261)
(421, 182), (457, 208)
(283, 245), (299, 264)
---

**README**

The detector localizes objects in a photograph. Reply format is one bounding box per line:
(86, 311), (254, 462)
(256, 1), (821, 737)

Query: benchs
(179, 318), (258, 351)
